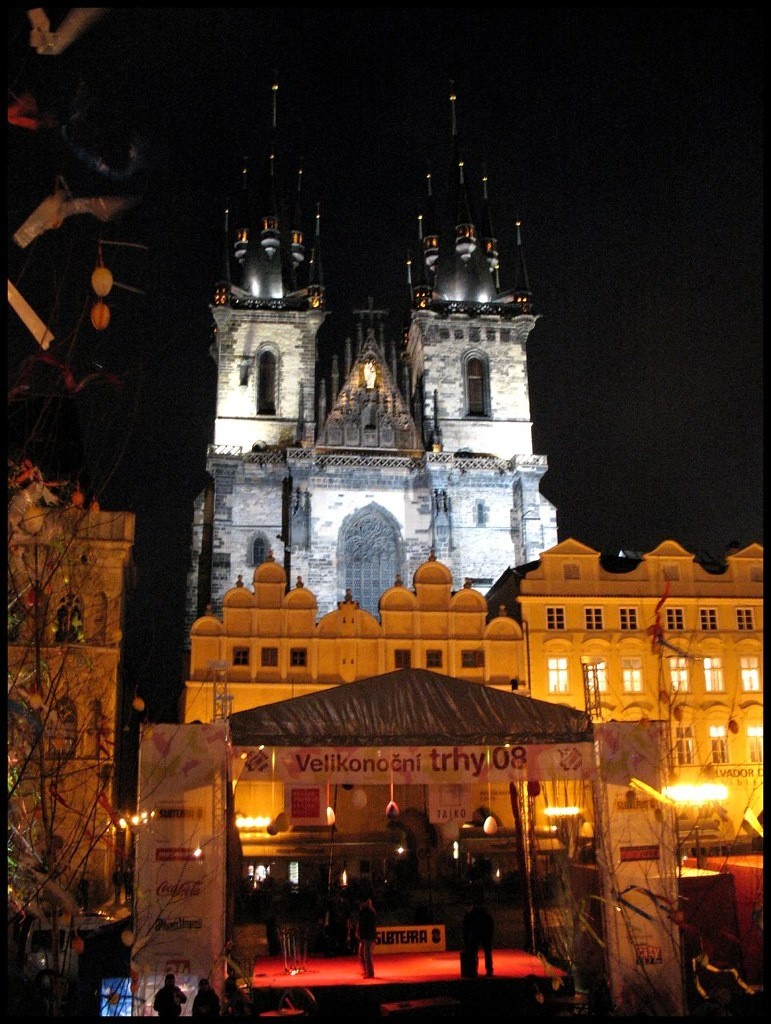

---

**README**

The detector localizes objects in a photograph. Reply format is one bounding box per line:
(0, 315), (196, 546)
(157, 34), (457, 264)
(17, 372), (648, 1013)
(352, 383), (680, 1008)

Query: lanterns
(72, 937), (84, 954)
(122, 931), (133, 946)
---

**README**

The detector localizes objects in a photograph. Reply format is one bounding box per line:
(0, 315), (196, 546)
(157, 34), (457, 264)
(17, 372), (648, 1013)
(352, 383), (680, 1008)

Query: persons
(459, 900), (494, 978)
(114, 866), (135, 902)
(192, 978), (221, 1016)
(355, 897), (379, 979)
(153, 974), (187, 1017)
(78, 874), (89, 906)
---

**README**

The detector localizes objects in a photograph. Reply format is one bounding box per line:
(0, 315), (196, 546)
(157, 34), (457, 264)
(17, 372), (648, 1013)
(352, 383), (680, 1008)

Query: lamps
(482, 745), (499, 835)
(386, 745), (400, 819)
(266, 747), (279, 836)
